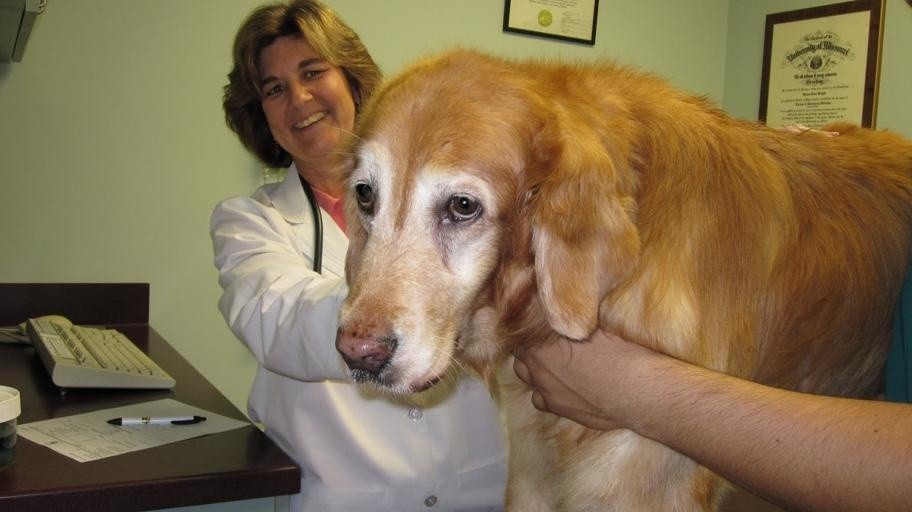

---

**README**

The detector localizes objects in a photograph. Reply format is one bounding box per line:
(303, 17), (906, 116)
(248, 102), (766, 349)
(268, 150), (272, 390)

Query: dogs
(315, 41), (912, 512)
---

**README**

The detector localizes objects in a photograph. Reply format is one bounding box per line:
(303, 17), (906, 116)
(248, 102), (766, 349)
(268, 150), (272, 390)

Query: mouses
(17, 315), (73, 336)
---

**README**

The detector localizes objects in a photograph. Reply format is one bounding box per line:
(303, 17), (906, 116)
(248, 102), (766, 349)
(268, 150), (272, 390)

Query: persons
(510, 261), (912, 512)
(208, 0), (507, 512)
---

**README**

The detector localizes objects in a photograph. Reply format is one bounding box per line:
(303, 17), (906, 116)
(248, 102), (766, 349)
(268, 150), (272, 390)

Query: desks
(0, 321), (300, 512)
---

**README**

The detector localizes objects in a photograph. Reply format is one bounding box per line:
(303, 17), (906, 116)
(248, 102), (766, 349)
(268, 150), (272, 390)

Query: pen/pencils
(106, 416), (207, 426)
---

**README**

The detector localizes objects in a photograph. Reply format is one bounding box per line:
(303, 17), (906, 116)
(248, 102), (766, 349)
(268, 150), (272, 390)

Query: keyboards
(25, 317), (178, 390)
